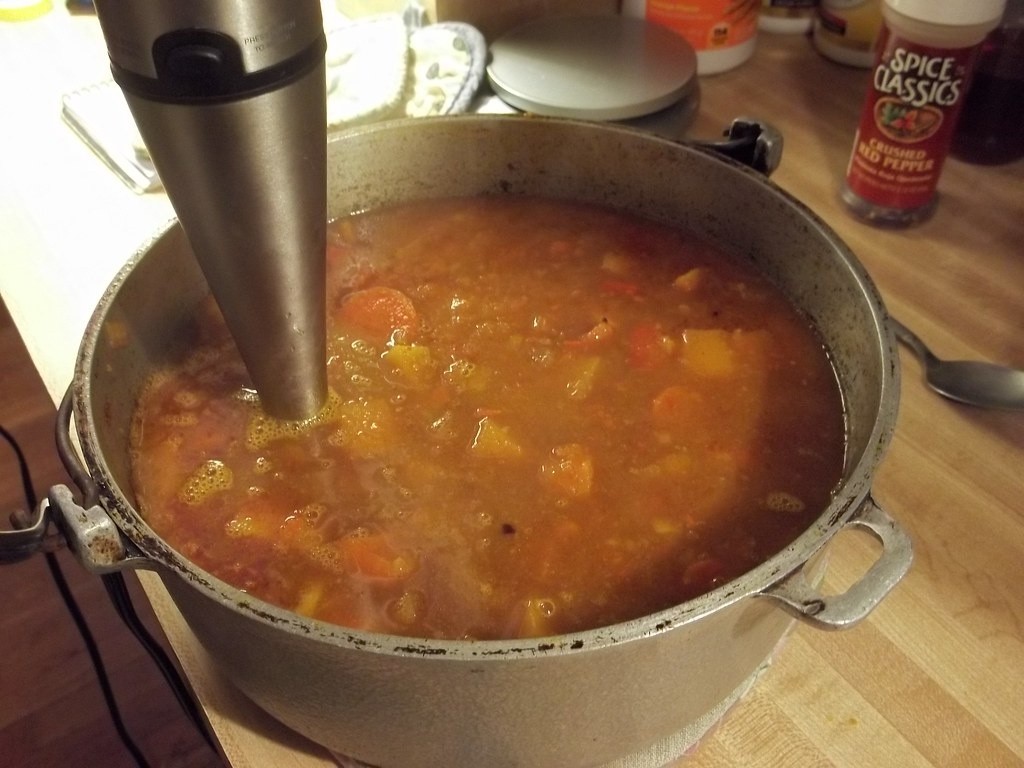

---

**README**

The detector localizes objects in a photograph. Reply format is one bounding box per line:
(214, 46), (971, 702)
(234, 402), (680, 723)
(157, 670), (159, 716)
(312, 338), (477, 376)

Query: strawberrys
(892, 110), (918, 130)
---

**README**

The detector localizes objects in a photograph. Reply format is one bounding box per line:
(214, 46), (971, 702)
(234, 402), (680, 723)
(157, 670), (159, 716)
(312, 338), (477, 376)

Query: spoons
(883, 311), (1024, 419)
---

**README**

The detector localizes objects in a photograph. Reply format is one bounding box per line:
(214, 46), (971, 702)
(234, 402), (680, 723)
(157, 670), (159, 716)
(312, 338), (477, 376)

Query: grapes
(881, 104), (907, 125)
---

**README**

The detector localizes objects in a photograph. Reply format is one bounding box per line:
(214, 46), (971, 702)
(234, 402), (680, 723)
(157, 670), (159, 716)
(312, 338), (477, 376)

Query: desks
(0, 0), (1024, 768)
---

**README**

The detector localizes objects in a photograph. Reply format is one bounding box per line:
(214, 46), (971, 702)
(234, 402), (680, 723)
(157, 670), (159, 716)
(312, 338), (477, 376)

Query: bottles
(841, 0), (1007, 231)
(614, 1), (879, 74)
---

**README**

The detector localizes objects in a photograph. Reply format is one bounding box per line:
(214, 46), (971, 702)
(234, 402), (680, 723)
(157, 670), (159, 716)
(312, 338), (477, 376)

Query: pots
(49, 118), (915, 768)
